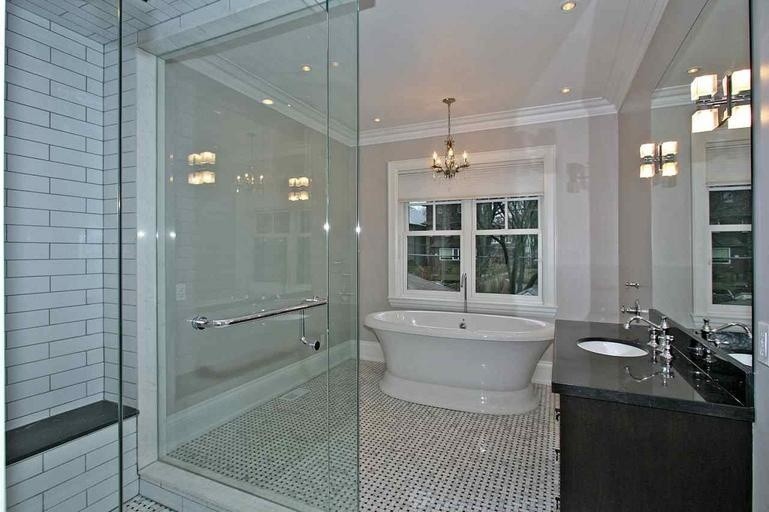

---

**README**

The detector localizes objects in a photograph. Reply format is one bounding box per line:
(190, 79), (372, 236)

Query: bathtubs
(360, 309), (556, 417)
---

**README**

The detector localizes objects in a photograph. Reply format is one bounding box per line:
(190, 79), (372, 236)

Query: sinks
(576, 337), (648, 358)
(727, 350), (754, 366)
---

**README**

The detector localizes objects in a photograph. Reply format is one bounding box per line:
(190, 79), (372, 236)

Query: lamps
(287, 178), (298, 201)
(431, 97), (470, 179)
(232, 132), (265, 195)
(187, 153), (202, 184)
(722, 69), (751, 131)
(690, 73), (719, 133)
(639, 143), (656, 179)
(298, 176), (311, 201)
(656, 141), (678, 177)
(200, 152), (216, 185)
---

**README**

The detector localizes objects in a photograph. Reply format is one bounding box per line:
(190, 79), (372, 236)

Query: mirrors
(649, 1), (753, 373)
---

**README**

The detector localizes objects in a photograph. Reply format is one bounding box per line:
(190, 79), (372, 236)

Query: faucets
(712, 321), (752, 341)
(622, 365), (668, 385)
(459, 270), (469, 312)
(623, 316), (659, 331)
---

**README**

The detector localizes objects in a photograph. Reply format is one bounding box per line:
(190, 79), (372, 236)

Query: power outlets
(757, 320), (768, 365)
